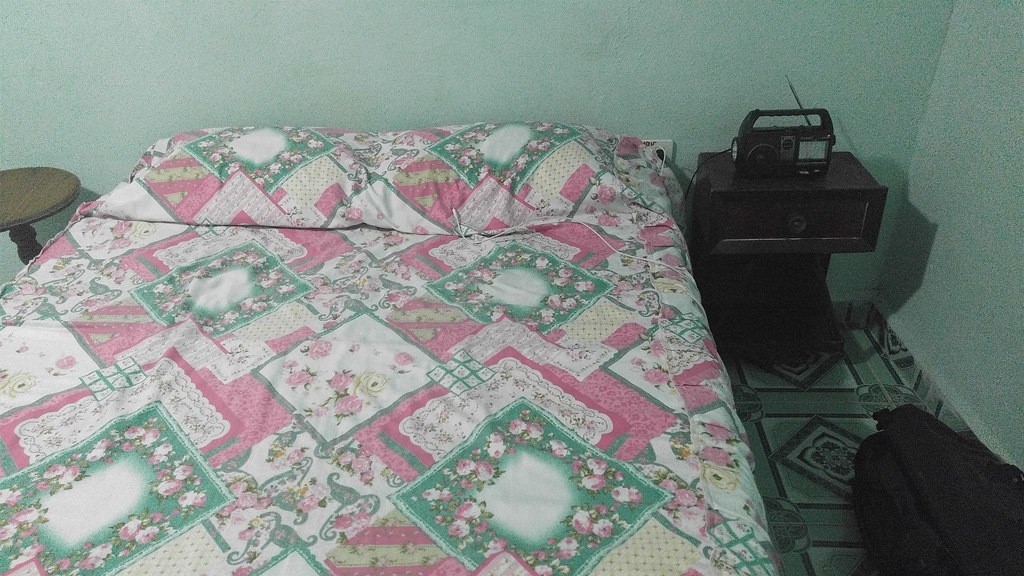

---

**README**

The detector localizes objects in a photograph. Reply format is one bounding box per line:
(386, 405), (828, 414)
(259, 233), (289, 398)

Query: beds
(0, 120), (781, 576)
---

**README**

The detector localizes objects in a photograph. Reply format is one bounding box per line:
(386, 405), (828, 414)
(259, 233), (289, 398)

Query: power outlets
(642, 140), (673, 166)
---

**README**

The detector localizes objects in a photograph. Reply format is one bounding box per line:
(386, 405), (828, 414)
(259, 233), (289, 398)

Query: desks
(0, 168), (83, 267)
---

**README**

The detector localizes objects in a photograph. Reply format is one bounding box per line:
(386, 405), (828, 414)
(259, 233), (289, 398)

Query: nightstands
(690, 152), (887, 351)
